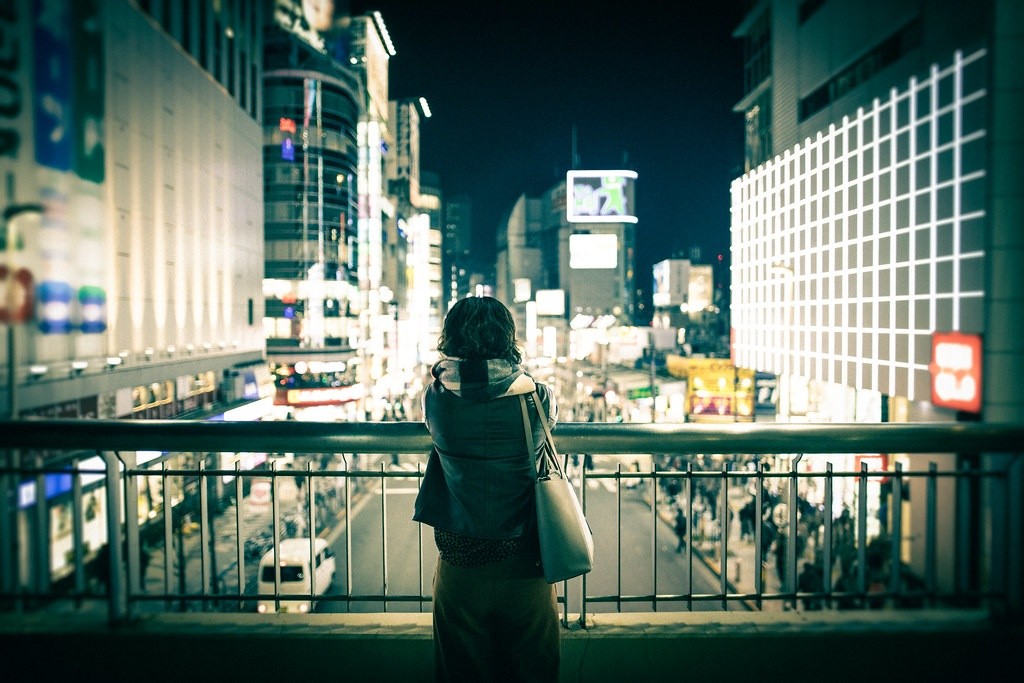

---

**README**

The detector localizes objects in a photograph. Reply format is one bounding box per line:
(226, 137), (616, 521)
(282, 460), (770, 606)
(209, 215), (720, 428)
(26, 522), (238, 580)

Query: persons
(570, 398), (940, 611)
(412, 296), (561, 683)
(269, 364), (316, 389)
(94, 536), (152, 594)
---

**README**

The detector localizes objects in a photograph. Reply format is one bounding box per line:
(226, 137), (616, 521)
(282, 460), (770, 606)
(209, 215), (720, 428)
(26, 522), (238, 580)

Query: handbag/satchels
(519, 390), (594, 584)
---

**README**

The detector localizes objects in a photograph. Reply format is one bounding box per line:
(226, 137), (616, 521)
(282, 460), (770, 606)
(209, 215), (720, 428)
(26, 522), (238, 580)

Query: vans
(258, 537), (336, 613)
